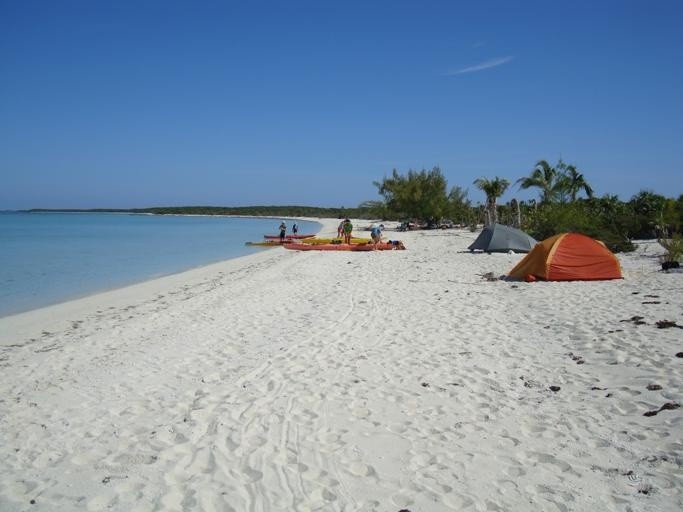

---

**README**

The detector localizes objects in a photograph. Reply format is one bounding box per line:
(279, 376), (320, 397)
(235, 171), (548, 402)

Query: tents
(468, 223), (537, 252)
(509, 232), (622, 281)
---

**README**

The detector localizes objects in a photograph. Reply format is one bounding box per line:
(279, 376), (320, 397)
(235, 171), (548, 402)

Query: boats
(244, 234), (393, 251)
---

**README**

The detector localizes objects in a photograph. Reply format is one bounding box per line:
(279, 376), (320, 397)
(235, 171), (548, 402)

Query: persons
(371, 227), (383, 244)
(279, 220), (287, 241)
(292, 223), (299, 235)
(338, 219), (353, 244)
